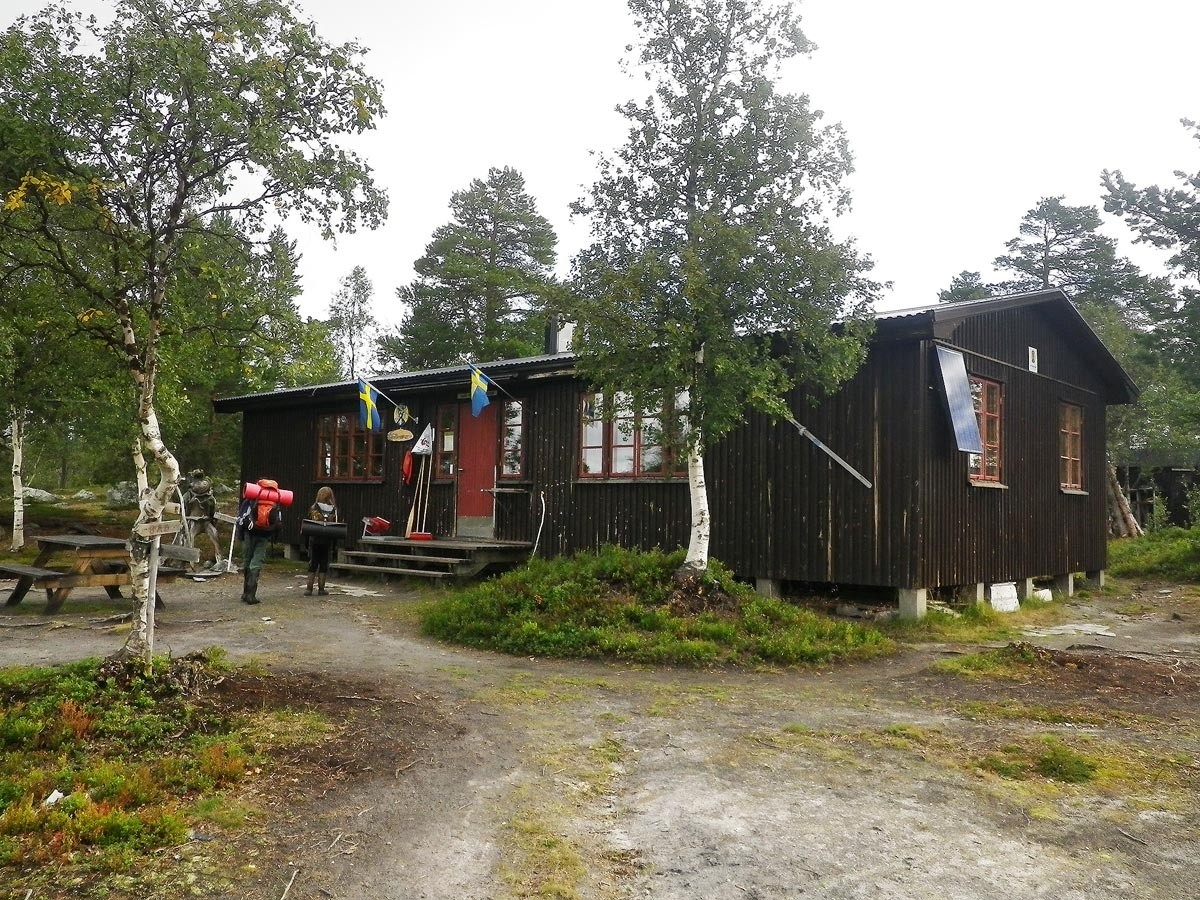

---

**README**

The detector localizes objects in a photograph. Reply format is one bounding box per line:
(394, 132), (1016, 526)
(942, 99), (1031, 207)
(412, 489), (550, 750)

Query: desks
(6, 535), (166, 616)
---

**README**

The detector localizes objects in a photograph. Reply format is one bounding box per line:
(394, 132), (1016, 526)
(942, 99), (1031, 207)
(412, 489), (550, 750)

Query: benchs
(108, 561), (186, 578)
(0, 565), (69, 583)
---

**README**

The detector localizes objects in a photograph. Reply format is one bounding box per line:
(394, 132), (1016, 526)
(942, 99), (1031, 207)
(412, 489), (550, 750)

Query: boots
(247, 573), (259, 605)
(241, 571), (249, 600)
(304, 572), (315, 596)
(318, 572), (329, 595)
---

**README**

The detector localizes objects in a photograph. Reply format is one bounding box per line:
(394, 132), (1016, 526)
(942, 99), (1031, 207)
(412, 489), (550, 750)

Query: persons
(300, 487), (348, 596)
(236, 475), (293, 605)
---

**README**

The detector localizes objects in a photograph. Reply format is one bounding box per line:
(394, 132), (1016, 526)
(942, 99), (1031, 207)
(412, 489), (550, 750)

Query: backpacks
(249, 499), (277, 530)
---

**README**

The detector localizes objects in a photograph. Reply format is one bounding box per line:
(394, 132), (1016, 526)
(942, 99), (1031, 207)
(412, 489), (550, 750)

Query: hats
(258, 480), (278, 492)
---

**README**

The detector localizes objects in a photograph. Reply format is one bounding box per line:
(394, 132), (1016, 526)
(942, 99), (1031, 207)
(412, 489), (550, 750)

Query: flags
(466, 361), (491, 418)
(358, 376), (381, 432)
